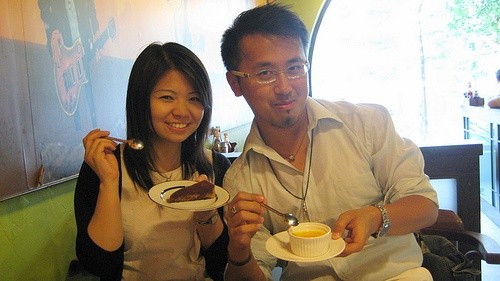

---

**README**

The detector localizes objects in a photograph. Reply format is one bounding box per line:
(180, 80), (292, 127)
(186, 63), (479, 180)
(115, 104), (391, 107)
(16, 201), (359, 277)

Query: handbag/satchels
(415, 234), (481, 281)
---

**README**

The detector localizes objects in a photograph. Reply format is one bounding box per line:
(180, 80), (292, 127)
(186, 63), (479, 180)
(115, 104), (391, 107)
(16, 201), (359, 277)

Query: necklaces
(156, 158), (178, 181)
(274, 130), (307, 160)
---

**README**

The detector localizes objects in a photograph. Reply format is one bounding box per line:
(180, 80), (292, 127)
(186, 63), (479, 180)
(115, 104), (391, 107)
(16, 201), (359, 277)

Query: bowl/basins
(288, 222), (331, 257)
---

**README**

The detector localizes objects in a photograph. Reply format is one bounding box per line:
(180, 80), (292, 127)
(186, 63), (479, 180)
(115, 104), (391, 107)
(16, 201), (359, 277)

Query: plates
(265, 231), (346, 263)
(148, 180), (230, 211)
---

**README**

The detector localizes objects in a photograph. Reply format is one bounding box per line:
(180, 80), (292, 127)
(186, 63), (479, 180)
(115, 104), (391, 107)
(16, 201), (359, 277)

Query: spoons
(260, 202), (300, 226)
(103, 136), (144, 150)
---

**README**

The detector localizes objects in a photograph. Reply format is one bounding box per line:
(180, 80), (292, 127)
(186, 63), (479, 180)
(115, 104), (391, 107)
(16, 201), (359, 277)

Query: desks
(461, 98), (500, 227)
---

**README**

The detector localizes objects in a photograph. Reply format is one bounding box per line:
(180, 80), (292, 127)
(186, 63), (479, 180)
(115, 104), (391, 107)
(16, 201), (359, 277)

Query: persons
(222, 5), (439, 281)
(74, 43), (233, 281)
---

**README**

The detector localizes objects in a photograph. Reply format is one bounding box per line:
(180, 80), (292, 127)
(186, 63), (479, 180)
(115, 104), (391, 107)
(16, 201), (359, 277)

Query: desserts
(168, 179), (215, 201)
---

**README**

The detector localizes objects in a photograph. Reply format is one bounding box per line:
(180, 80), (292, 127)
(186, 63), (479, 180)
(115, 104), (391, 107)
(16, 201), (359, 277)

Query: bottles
(463, 81), (479, 98)
(211, 125), (232, 153)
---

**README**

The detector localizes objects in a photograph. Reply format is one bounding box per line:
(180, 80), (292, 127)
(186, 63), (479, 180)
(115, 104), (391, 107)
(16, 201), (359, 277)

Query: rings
(232, 205), (237, 214)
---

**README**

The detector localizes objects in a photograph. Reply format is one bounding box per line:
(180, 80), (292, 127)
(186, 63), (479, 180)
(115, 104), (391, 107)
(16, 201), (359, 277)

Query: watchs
(196, 211), (218, 224)
(370, 202), (390, 239)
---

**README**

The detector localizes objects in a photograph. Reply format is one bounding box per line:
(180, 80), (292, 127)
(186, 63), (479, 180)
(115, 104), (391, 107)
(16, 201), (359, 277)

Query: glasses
(228, 59), (310, 85)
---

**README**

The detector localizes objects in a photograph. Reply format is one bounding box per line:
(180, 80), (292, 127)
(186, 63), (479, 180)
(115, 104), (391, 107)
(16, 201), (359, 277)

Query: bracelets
(229, 254), (251, 266)
